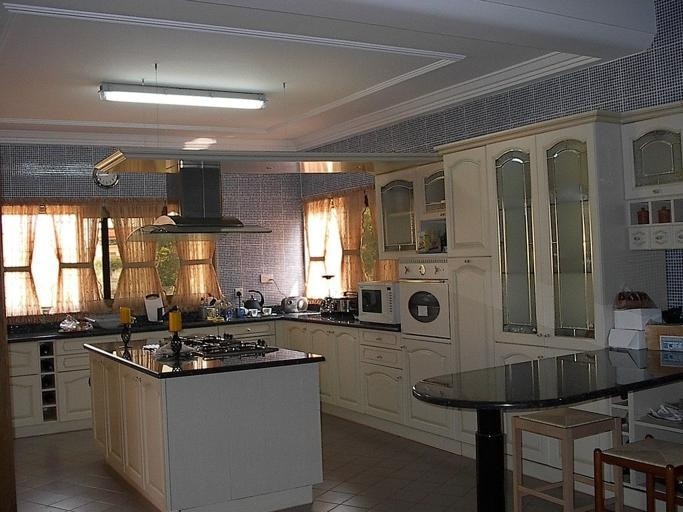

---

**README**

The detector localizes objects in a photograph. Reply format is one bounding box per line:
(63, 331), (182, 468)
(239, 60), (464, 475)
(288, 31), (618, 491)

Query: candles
(118, 304), (131, 324)
(168, 310), (181, 332)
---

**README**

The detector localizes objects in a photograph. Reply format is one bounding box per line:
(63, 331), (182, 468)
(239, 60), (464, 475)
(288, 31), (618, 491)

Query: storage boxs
(607, 306), (683, 352)
(608, 351), (683, 386)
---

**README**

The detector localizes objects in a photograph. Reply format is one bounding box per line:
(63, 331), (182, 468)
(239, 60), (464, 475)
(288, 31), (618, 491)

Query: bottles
(199, 294), (214, 305)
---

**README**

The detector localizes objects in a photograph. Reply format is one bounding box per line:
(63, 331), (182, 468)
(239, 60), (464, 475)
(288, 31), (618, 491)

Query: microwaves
(358, 282), (401, 325)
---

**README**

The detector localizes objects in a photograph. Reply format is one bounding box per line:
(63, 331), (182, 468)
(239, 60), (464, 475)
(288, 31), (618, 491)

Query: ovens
(400, 278), (450, 341)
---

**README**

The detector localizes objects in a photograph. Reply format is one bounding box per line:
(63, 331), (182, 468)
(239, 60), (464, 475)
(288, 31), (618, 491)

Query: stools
(510, 406), (683, 512)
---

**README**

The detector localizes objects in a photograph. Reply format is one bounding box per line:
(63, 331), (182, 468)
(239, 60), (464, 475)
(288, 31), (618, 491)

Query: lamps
(153, 66), (177, 226)
(96, 81), (267, 110)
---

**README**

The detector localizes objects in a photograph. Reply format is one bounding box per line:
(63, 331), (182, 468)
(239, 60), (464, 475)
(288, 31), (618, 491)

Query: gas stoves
(161, 334), (279, 360)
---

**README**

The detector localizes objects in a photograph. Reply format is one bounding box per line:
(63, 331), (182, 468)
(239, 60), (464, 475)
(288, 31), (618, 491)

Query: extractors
(125, 160), (272, 242)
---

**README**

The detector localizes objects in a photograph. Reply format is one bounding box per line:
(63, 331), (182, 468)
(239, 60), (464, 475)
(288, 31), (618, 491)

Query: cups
(207, 308), (215, 317)
(262, 307), (271, 315)
(237, 307), (249, 317)
(249, 309), (259, 317)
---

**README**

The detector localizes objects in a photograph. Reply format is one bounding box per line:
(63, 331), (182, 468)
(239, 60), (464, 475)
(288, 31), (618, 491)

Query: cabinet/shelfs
(622, 100), (683, 251)
(7, 334), (120, 438)
(375, 160), (446, 260)
(89, 352), (170, 512)
(612, 379), (683, 511)
(219, 319), (276, 346)
(285, 322), (461, 456)
(503, 353), (605, 404)
(434, 109), (671, 495)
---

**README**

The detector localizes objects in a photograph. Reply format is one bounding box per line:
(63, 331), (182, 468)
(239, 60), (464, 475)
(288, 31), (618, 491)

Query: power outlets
(235, 287), (243, 298)
(259, 274), (273, 282)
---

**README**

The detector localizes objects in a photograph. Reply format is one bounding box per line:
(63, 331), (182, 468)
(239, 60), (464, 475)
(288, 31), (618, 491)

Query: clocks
(92, 167), (120, 188)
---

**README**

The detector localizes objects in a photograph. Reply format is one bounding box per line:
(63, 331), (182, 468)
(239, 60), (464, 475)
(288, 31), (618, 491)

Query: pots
(336, 298), (355, 321)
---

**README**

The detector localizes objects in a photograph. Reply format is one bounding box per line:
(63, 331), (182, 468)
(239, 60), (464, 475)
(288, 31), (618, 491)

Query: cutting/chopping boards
(143, 291), (165, 323)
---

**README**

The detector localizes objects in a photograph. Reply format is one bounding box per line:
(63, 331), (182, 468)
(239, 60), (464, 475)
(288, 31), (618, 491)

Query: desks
(412, 348), (683, 512)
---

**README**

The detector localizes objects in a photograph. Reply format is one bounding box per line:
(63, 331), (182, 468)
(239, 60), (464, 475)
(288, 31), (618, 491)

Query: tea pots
(242, 289), (264, 313)
